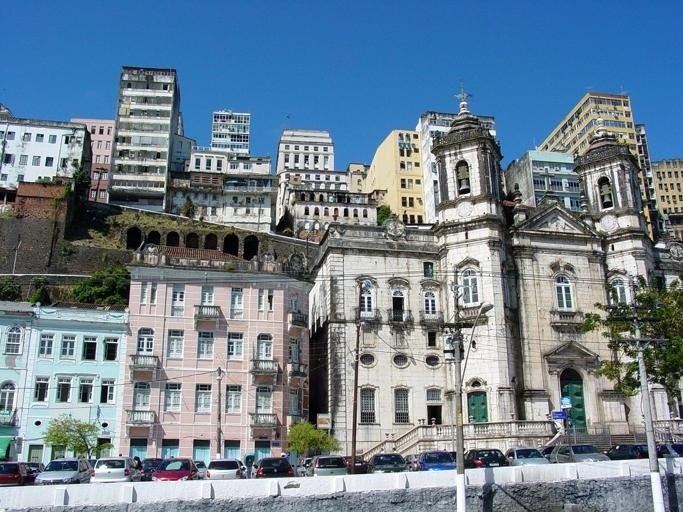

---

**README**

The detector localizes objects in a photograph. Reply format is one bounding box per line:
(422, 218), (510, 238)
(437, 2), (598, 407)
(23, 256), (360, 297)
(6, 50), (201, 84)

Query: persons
(380, 447), (384, 453)
(132, 456), (146, 481)
(391, 447), (396, 453)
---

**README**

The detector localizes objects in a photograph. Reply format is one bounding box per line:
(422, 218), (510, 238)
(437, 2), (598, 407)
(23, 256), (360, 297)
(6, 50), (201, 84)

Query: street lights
(452, 302), (493, 512)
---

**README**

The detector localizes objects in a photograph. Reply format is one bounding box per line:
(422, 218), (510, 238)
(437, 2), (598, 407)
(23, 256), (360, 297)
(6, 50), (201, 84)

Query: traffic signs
(550, 396), (575, 426)
